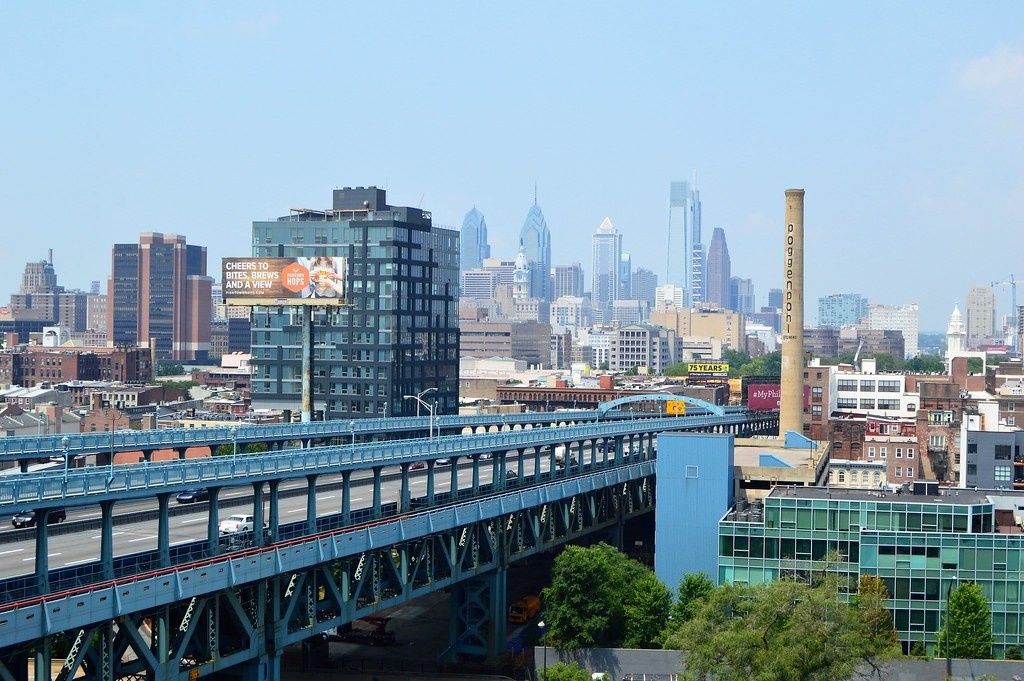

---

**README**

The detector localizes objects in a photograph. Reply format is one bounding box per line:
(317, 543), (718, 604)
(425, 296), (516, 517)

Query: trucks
(321, 616), (396, 646)
(508, 595), (541, 623)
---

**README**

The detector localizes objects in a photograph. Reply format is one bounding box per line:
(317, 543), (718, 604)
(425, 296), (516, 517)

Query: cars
(598, 439), (615, 452)
(498, 469), (518, 481)
(625, 445), (641, 455)
(397, 452), (501, 472)
(218, 515), (267, 534)
(544, 442), (578, 470)
(176, 487), (210, 504)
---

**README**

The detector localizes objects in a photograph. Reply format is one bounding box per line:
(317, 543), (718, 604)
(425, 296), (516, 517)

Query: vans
(11, 507), (66, 529)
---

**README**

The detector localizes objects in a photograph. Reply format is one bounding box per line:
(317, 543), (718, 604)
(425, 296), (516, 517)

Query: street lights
(155, 408), (161, 430)
(545, 400), (549, 413)
(349, 421), (356, 454)
(230, 426), (238, 465)
(434, 400), (438, 418)
(659, 406), (663, 418)
(403, 395), (433, 445)
(659, 390), (679, 418)
(553, 411), (558, 429)
(514, 400), (516, 414)
(477, 400), (480, 415)
(37, 410), (44, 437)
(436, 416), (442, 445)
(539, 619), (546, 681)
(323, 402), (328, 421)
(417, 388), (438, 418)
(60, 435), (71, 499)
(629, 407), (634, 421)
(596, 409), (599, 425)
(248, 405), (255, 423)
(573, 400), (577, 413)
(501, 414), (505, 434)
(384, 402), (388, 417)
